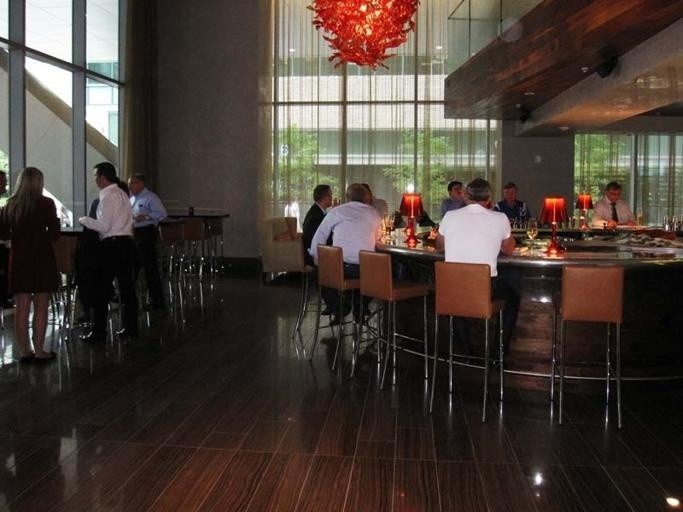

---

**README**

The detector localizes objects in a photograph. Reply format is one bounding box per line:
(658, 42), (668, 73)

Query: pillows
(272, 229), (297, 242)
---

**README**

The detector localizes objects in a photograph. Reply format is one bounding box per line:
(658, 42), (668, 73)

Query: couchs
(261, 216), (305, 286)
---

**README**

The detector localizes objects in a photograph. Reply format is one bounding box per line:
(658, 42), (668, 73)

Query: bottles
(662, 217), (683, 232)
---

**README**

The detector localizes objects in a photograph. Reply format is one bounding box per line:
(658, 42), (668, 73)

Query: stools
(349, 250), (430, 391)
(292, 233), (318, 340)
(550, 264), (625, 429)
(309, 244), (361, 371)
(430, 260), (507, 422)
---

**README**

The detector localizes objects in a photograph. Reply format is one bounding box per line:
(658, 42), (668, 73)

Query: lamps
(573, 192), (596, 232)
(538, 197), (570, 256)
(595, 54), (618, 79)
(306, 0), (423, 77)
(396, 194), (425, 243)
(517, 104), (532, 124)
(397, 194), (426, 235)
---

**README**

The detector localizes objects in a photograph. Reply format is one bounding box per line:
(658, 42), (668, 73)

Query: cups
(383, 212), (395, 236)
(636, 210), (642, 227)
(513, 216), (576, 229)
(188, 207), (192, 214)
(526, 218), (537, 240)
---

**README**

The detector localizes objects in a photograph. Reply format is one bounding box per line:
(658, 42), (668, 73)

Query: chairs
(0, 205), (232, 375)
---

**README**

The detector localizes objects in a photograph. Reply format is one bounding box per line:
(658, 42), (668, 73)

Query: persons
(310, 183), (382, 327)
(441, 181), (467, 219)
(493, 182), (530, 228)
(2, 167), (71, 363)
(302, 184), (341, 266)
(590, 181), (637, 229)
(361, 183), (388, 219)
(73, 162), (169, 343)
(284, 189), (301, 232)
(435, 177), (521, 370)
(0, 170), (16, 309)
